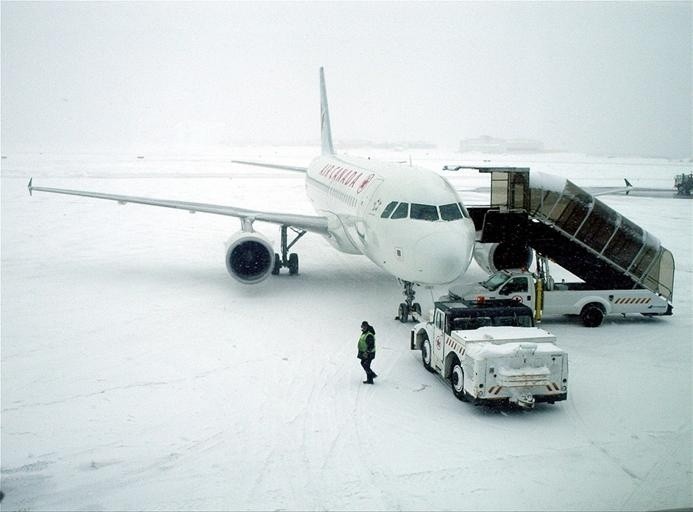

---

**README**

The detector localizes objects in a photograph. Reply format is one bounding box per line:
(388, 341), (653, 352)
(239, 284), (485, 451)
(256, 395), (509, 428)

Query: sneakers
(362, 373), (377, 385)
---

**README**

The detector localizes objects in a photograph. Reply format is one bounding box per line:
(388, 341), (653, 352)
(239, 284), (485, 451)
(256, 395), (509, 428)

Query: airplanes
(28, 67), (633, 323)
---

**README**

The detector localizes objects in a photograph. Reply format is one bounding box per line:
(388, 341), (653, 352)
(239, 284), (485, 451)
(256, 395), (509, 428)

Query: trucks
(411, 295), (569, 411)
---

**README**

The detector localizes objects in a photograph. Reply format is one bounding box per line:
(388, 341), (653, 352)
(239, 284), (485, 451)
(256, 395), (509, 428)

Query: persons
(356, 320), (377, 384)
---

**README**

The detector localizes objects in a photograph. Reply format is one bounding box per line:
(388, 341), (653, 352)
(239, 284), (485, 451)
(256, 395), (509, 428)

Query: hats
(360, 320), (368, 327)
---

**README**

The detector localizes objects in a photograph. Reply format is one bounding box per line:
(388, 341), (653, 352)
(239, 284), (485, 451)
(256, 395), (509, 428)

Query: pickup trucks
(448, 268), (673, 327)
